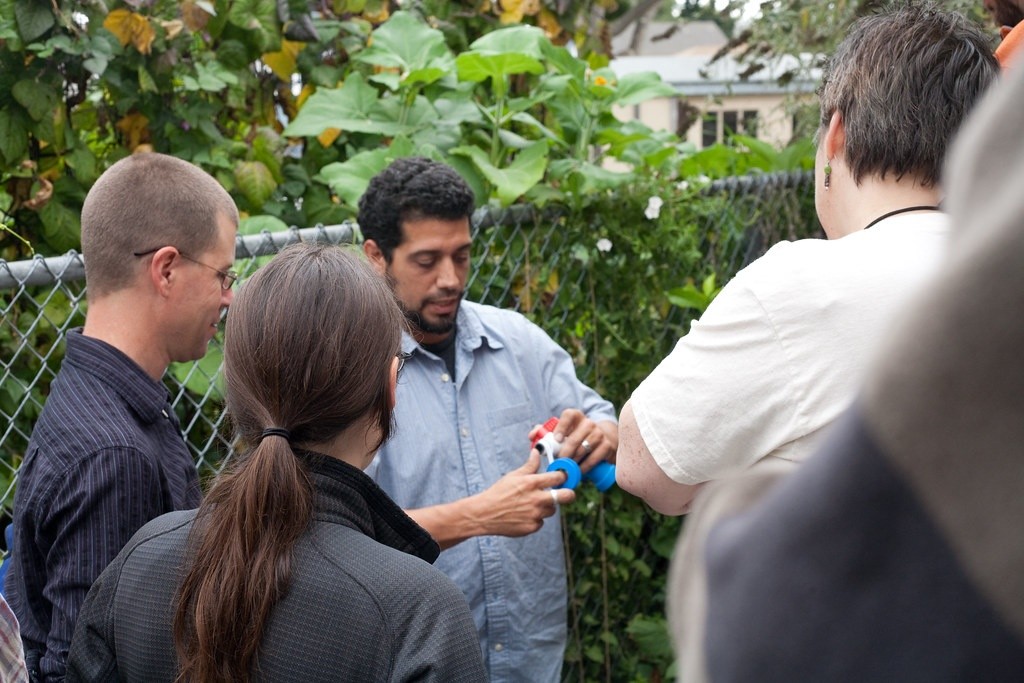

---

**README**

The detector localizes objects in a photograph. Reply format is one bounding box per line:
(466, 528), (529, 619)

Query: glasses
(134, 248), (236, 290)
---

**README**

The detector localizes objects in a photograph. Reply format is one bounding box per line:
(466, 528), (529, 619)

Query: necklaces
(863, 205), (942, 231)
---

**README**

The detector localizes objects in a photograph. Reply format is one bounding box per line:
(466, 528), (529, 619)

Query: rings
(581, 439), (592, 453)
(551, 489), (557, 505)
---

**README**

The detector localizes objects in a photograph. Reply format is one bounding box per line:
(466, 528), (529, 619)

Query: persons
(3, 151), (239, 683)
(614, 0), (1024, 683)
(62, 239), (491, 683)
(356, 156), (620, 683)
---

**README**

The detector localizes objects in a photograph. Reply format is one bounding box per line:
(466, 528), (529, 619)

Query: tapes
(547, 457), (582, 490)
